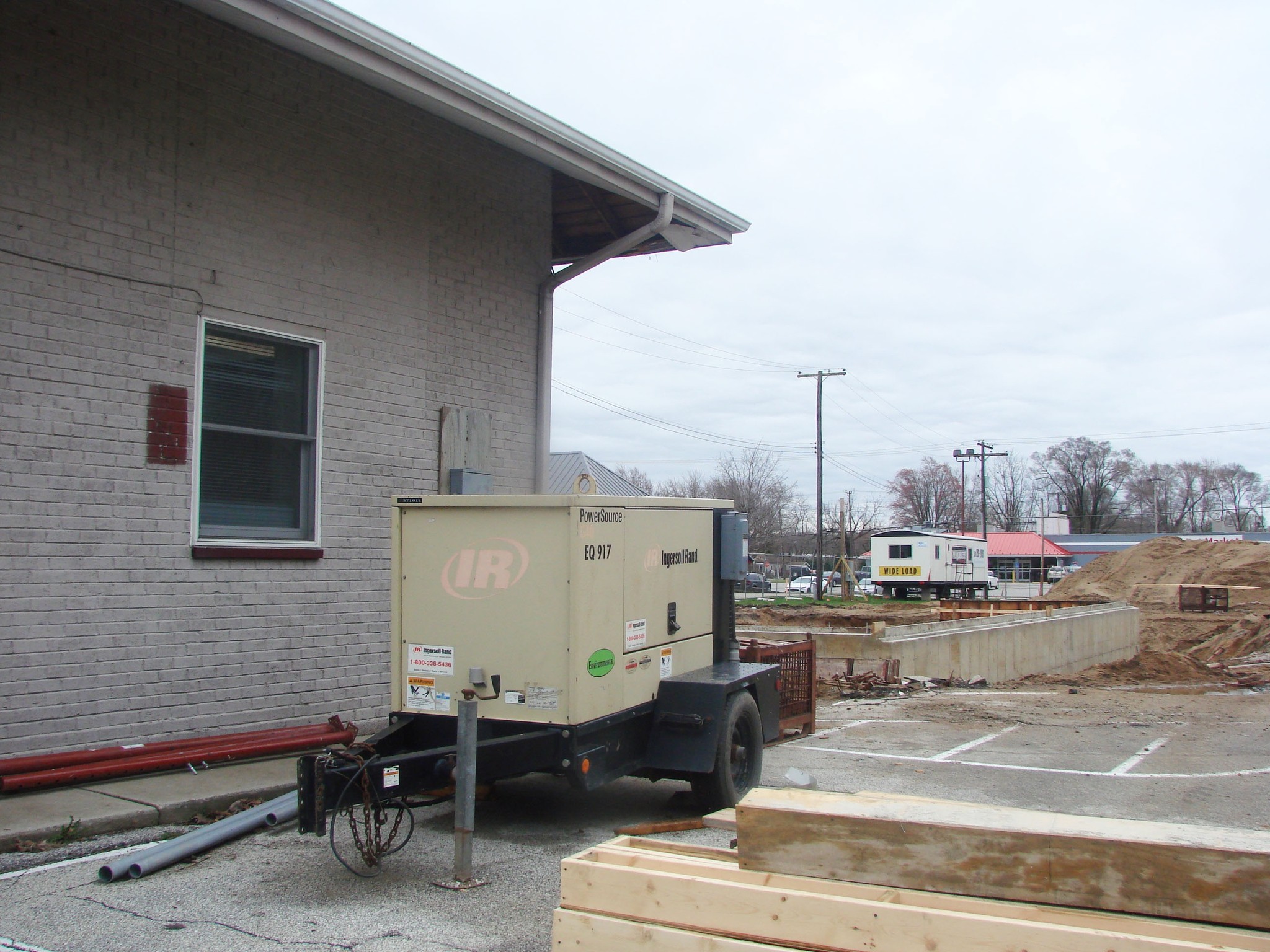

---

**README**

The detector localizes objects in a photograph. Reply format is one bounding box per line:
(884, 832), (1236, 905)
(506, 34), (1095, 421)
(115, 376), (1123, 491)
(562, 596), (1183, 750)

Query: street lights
(953, 449), (975, 536)
(1146, 474), (1166, 533)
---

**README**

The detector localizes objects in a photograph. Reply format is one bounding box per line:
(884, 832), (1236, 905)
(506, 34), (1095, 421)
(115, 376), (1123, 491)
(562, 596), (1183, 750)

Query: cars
(822, 571), (842, 587)
(734, 573), (772, 591)
(987, 570), (999, 590)
(785, 576), (830, 593)
(854, 578), (884, 595)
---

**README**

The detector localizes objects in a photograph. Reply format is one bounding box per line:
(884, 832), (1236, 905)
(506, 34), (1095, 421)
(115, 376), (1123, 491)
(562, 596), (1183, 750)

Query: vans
(860, 565), (871, 578)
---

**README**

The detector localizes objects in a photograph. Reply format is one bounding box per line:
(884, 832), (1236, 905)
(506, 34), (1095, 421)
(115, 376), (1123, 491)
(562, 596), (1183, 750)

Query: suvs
(785, 564), (817, 581)
(1047, 565), (1068, 584)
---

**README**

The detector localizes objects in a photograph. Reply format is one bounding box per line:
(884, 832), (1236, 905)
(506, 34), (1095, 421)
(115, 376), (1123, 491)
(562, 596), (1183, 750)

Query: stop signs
(764, 562), (769, 568)
